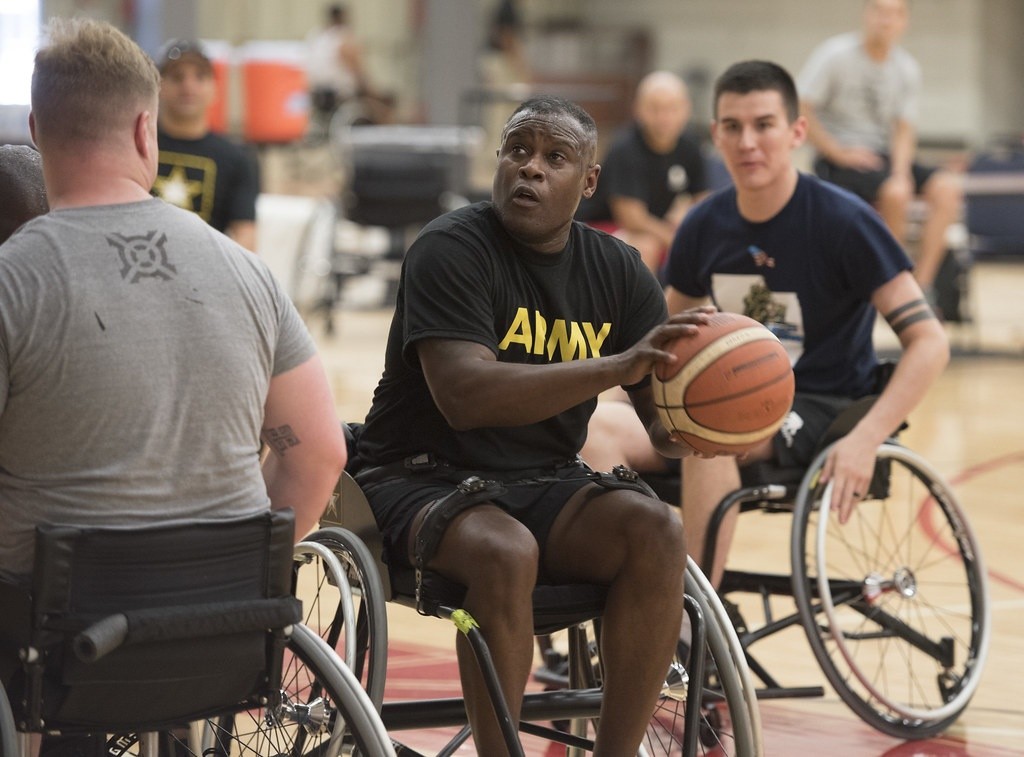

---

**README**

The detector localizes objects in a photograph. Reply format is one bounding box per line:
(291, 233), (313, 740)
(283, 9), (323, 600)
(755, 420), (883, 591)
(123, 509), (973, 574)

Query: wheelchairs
(214, 421), (759, 757)
(0, 508), (397, 757)
(637, 355), (987, 738)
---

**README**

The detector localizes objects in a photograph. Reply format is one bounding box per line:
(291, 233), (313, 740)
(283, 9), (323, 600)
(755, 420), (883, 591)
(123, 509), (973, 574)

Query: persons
(305, 5), (393, 118)
(474, 2), (541, 87)
(531, 61), (950, 686)
(351, 99), (718, 757)
(794, 0), (963, 292)
(0, 16), (347, 756)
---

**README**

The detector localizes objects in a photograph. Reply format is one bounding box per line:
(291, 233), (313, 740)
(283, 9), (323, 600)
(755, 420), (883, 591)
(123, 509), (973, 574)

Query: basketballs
(651, 312), (796, 454)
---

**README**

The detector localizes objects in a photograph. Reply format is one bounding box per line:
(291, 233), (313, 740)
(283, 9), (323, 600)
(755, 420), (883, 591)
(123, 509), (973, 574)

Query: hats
(155, 39), (210, 68)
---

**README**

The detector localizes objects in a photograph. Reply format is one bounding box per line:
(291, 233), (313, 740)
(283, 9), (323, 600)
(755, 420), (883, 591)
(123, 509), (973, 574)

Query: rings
(853, 491), (861, 499)
